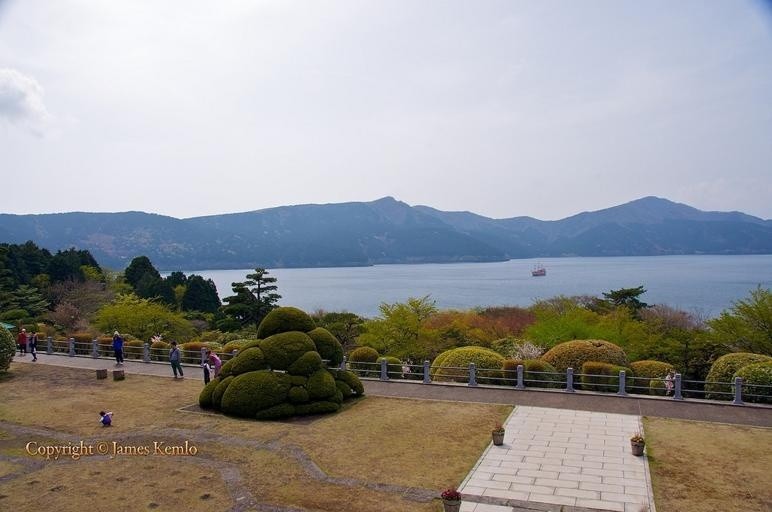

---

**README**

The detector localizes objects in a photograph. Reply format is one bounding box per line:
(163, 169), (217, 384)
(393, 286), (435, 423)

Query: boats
(532, 263), (546, 276)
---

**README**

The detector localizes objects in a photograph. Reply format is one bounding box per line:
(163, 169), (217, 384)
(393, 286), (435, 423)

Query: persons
(206, 351), (221, 376)
(204, 359), (212, 386)
(111, 330), (125, 365)
(17, 328), (27, 354)
(169, 340), (185, 378)
(97, 410), (114, 426)
(29, 331), (38, 362)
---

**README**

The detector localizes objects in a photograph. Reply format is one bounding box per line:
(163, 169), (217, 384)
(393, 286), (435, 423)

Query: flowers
(440, 488), (460, 501)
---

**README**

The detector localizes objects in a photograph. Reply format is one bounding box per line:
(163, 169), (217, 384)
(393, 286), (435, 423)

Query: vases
(442, 499), (460, 511)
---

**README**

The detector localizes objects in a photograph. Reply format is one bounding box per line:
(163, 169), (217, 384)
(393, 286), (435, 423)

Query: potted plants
(630, 434), (645, 456)
(491, 422), (504, 445)
